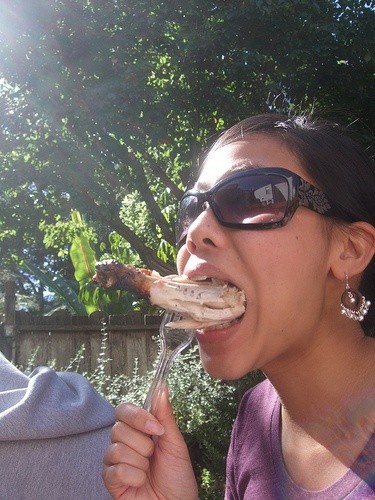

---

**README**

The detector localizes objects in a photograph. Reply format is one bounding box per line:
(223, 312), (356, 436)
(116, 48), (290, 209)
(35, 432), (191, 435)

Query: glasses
(176, 167), (358, 249)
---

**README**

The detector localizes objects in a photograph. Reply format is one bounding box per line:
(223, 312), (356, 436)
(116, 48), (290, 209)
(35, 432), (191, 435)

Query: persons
(101, 112), (375, 500)
(1, 350), (116, 500)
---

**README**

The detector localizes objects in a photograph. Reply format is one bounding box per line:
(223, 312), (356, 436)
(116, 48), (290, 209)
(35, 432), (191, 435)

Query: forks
(142, 307), (196, 412)
